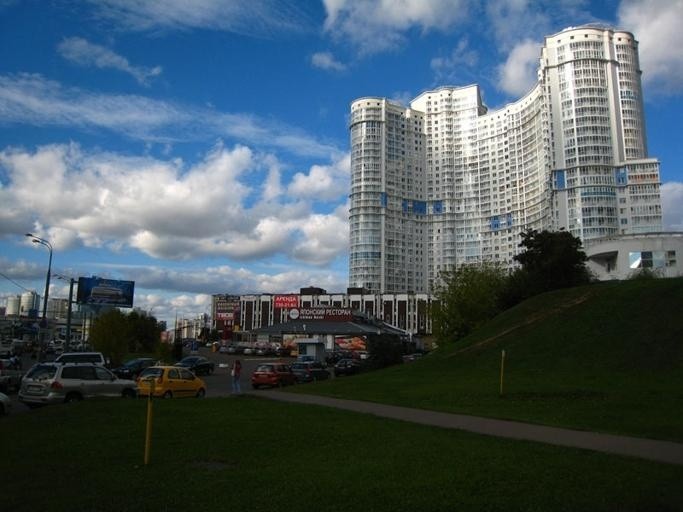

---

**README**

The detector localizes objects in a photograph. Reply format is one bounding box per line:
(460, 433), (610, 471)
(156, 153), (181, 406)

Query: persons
(229, 359), (241, 393)
(0, 350), (25, 395)
(38, 342), (55, 363)
(127, 364), (144, 380)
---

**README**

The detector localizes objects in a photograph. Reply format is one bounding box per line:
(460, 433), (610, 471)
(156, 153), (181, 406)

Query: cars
(1, 335), (139, 413)
(334, 358), (361, 375)
(173, 356), (215, 376)
(137, 365), (207, 399)
(292, 361), (331, 383)
(218, 345), (299, 356)
(112, 357), (157, 379)
(249, 362), (298, 388)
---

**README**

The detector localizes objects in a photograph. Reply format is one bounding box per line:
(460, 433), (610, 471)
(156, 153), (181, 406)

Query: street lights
(24, 232), (53, 360)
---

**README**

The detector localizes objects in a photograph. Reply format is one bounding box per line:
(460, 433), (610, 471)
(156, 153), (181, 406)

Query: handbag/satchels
(231, 370), (235, 376)
